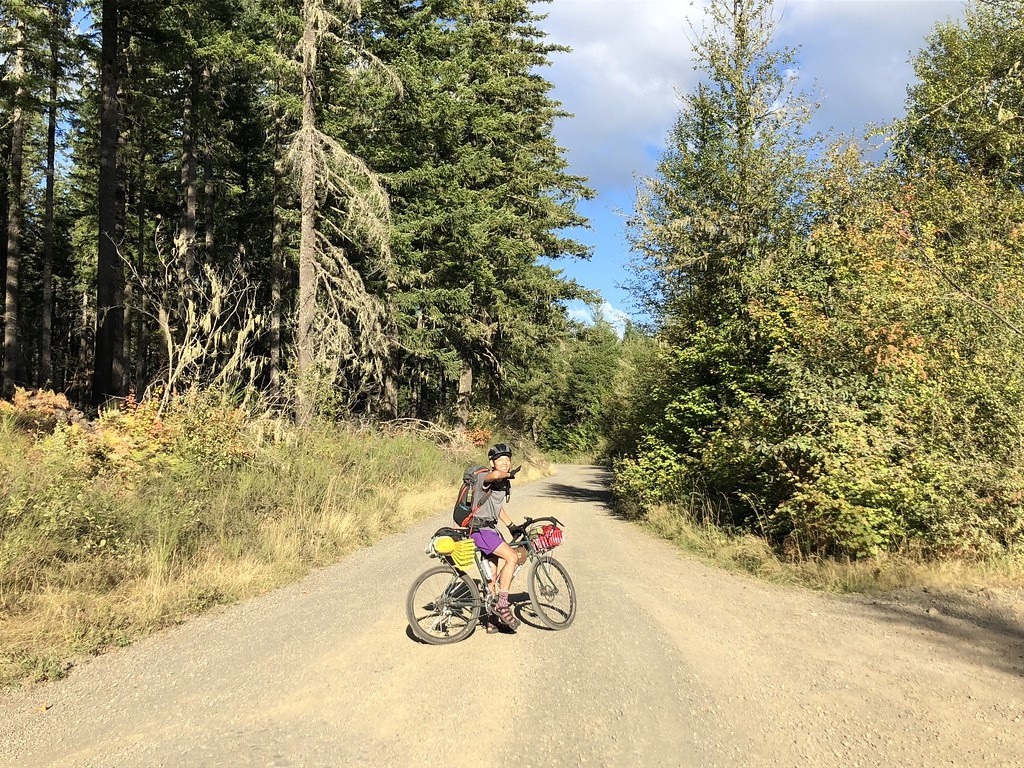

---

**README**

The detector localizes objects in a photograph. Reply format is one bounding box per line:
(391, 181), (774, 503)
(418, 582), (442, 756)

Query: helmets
(488, 443), (512, 460)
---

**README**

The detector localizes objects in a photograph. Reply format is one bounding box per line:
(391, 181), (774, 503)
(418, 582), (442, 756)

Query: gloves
(506, 466), (521, 479)
(506, 522), (522, 537)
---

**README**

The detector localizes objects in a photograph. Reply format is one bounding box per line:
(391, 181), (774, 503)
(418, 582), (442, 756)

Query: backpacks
(453, 466), (494, 526)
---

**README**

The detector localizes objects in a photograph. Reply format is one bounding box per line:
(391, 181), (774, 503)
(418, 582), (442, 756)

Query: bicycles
(405, 516), (577, 646)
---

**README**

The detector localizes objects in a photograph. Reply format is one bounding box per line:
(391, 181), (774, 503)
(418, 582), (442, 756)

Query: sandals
(493, 605), (518, 627)
(485, 621), (510, 632)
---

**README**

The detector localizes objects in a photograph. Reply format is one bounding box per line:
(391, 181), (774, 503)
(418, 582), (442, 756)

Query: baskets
(530, 530), (563, 553)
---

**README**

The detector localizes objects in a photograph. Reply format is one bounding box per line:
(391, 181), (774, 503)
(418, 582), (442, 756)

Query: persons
(471, 443), (521, 634)
